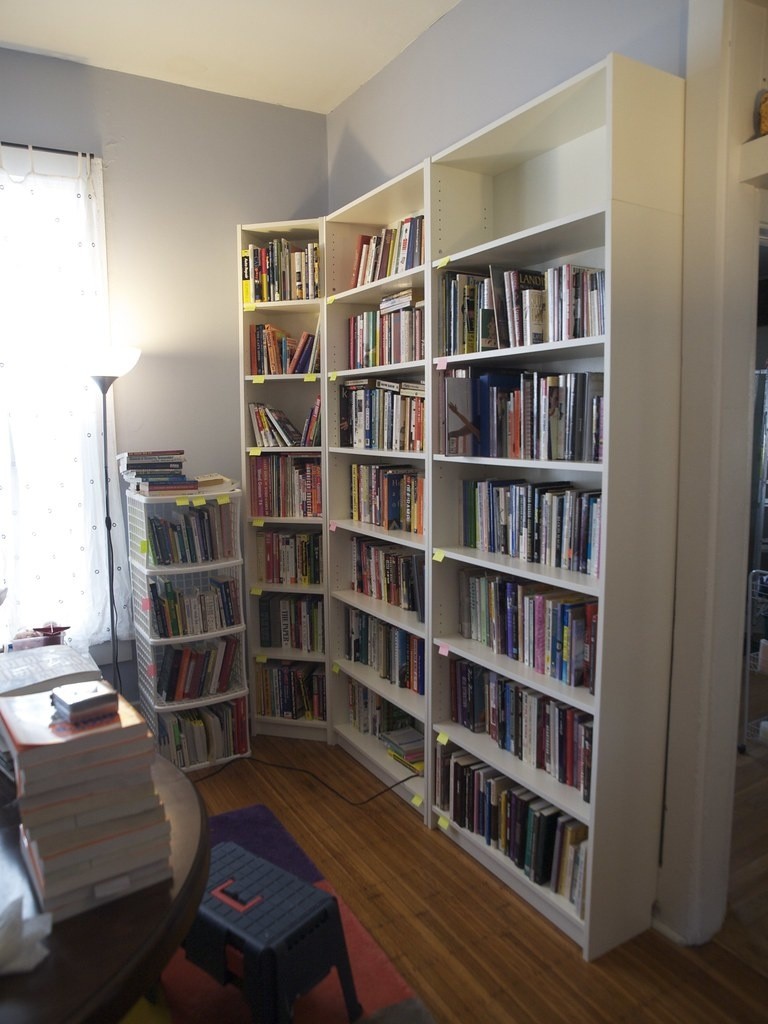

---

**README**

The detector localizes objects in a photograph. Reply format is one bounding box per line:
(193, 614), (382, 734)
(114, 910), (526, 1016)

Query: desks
(0, 752), (211, 1024)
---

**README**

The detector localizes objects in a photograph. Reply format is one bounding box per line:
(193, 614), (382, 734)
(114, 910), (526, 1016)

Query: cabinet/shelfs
(125, 52), (686, 965)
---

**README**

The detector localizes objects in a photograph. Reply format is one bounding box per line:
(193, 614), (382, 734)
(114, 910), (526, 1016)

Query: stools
(181, 841), (364, 1024)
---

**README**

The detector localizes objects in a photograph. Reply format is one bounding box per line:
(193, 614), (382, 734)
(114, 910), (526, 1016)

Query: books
(349, 289), (424, 369)
(242, 238), (319, 304)
(349, 215), (425, 288)
(438, 262), (605, 355)
(250, 314), (320, 374)
(116, 448), (247, 768)
(0, 645), (173, 924)
(248, 367), (604, 917)
